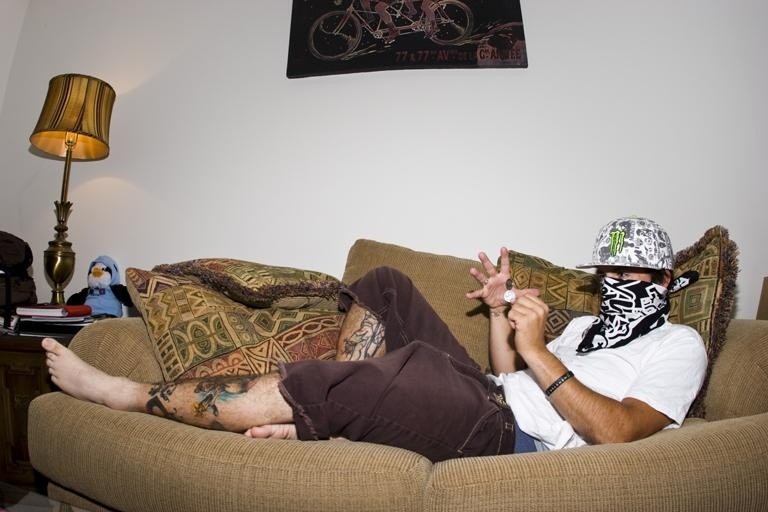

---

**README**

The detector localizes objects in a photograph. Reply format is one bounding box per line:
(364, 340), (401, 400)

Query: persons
(359, 0), (399, 43)
(404, 0), (440, 39)
(40, 216), (711, 465)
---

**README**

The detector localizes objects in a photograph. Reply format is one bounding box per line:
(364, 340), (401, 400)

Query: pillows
(126, 267), (347, 385)
(341, 238), (492, 379)
(496, 224), (741, 419)
(151, 257), (345, 309)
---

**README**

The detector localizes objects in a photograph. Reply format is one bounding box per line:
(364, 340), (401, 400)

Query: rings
(481, 279), (488, 285)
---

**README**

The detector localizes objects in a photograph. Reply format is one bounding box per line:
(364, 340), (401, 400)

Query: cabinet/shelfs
(0, 334), (74, 485)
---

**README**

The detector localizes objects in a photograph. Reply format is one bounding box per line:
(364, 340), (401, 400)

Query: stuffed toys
(66, 256), (133, 319)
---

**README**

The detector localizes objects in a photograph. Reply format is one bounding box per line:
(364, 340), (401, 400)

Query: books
(15, 304), (98, 338)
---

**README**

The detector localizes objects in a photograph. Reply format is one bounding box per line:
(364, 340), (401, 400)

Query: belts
(485, 381), (516, 456)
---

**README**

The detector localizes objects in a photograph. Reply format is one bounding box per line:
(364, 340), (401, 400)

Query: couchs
(27, 237), (767, 511)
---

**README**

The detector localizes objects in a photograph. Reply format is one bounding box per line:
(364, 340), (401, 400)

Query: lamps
(28, 73), (116, 309)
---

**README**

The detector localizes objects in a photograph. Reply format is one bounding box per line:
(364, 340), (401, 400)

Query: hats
(576, 214), (675, 273)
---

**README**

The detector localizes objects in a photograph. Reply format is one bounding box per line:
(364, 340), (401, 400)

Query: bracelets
(545, 371), (574, 396)
